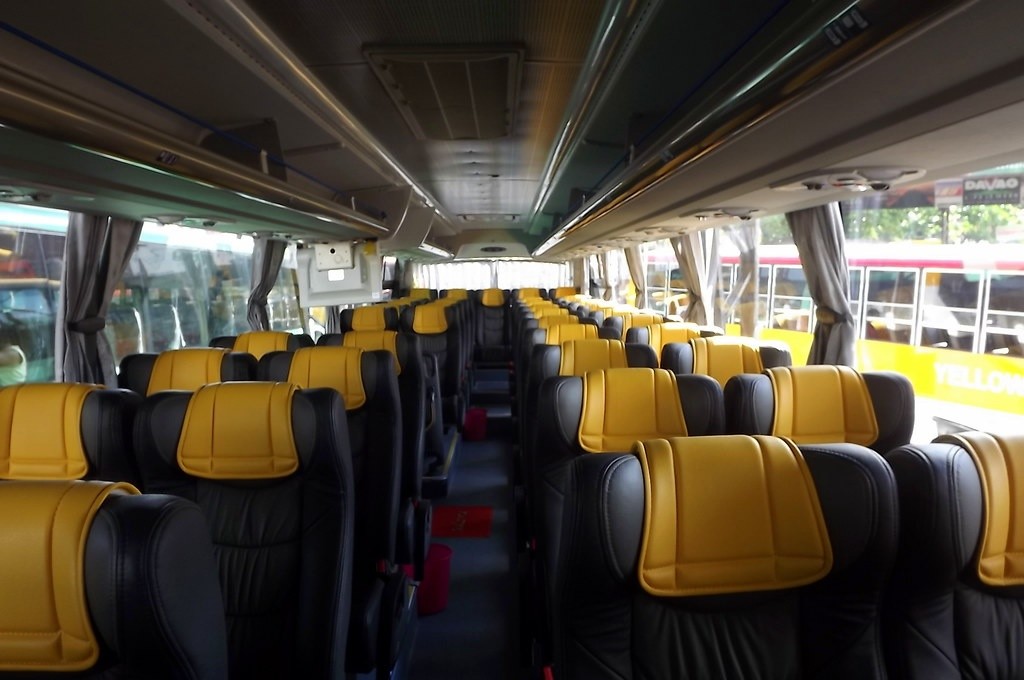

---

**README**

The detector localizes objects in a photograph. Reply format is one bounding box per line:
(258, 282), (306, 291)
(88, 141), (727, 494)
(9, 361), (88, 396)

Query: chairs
(0, 281), (1024, 680)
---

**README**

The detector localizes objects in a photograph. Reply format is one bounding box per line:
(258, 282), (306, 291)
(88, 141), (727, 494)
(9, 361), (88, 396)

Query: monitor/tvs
(297, 242), (384, 309)
